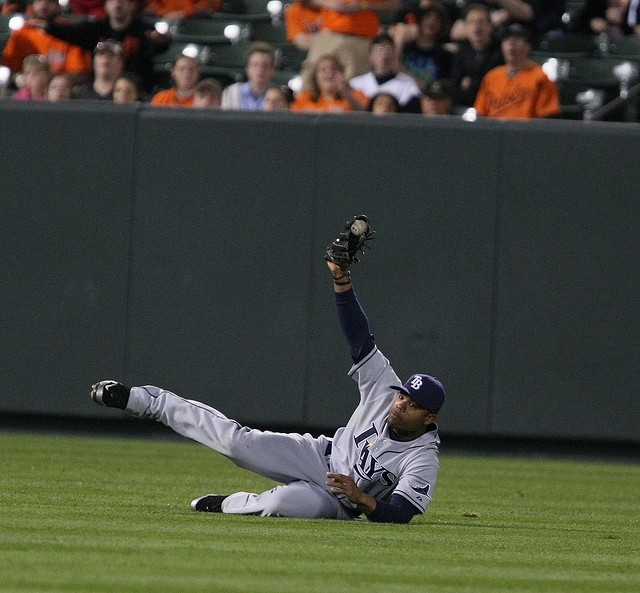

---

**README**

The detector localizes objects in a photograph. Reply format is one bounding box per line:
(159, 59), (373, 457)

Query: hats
(390, 374), (445, 415)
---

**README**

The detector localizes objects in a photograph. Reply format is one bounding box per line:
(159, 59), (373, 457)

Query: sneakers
(91, 380), (131, 410)
(191, 494), (228, 512)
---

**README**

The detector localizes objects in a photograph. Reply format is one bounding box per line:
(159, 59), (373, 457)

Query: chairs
(430, 0), (640, 122)
(69, 0), (304, 108)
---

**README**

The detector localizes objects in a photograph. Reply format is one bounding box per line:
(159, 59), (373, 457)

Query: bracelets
(334, 277), (352, 286)
(333, 270), (351, 280)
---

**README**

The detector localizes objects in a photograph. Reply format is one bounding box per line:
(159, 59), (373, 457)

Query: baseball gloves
(321, 215), (374, 267)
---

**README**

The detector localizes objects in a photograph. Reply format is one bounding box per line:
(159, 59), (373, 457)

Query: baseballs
(348, 219), (368, 237)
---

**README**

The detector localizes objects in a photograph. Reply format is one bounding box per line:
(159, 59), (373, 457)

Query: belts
(325, 440), (332, 470)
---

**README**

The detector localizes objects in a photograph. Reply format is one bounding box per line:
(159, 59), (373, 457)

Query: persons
(221, 43), (275, 111)
(2, 1), (224, 112)
(266, 0), (640, 118)
(91, 261), (446, 524)
